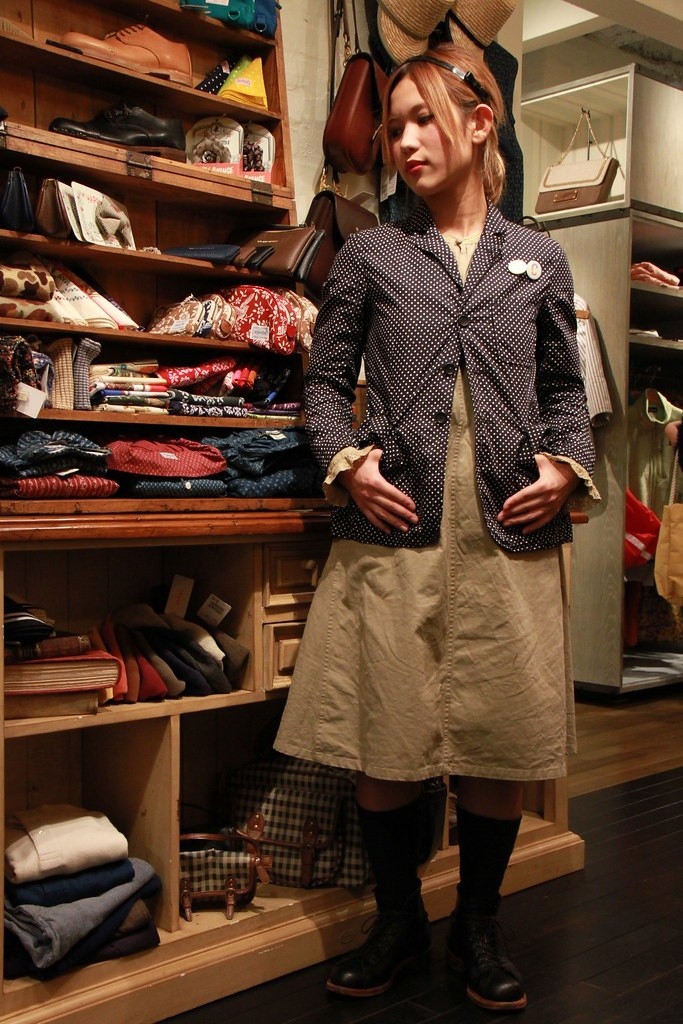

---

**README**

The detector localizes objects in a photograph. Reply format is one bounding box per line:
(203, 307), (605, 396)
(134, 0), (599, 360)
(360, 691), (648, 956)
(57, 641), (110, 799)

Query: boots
(446, 884), (529, 1013)
(324, 878), (433, 998)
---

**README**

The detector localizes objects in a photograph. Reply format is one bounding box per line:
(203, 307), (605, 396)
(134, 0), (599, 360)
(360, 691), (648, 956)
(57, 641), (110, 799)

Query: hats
(447, 0), (518, 48)
(376, 0), (455, 69)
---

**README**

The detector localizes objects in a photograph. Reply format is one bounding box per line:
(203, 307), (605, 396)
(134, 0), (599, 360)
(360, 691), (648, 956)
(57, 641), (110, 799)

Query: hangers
(629, 366), (642, 399)
(646, 365), (663, 406)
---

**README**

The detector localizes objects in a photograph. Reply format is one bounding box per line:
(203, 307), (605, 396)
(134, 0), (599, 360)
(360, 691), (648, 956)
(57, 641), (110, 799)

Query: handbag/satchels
(145, 284), (320, 356)
(534, 109), (619, 214)
(0, 165), (35, 233)
(180, 0), (282, 40)
(306, 189), (380, 286)
(226, 754), (369, 891)
(654, 503), (683, 606)
(179, 833), (270, 922)
(33, 175), (71, 238)
(322, 51), (389, 184)
(158, 225), (327, 281)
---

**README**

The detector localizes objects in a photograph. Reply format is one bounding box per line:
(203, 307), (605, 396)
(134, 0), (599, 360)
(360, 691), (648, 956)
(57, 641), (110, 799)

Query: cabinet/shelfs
(0, 0), (588, 1024)
(517, 60), (683, 804)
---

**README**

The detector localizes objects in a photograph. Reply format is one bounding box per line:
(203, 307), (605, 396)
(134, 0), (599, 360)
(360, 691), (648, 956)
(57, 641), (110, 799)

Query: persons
(271, 47), (601, 1008)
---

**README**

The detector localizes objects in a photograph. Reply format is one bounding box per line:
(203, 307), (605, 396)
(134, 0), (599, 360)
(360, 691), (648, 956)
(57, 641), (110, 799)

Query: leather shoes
(48, 100), (187, 164)
(60, 23), (194, 88)
